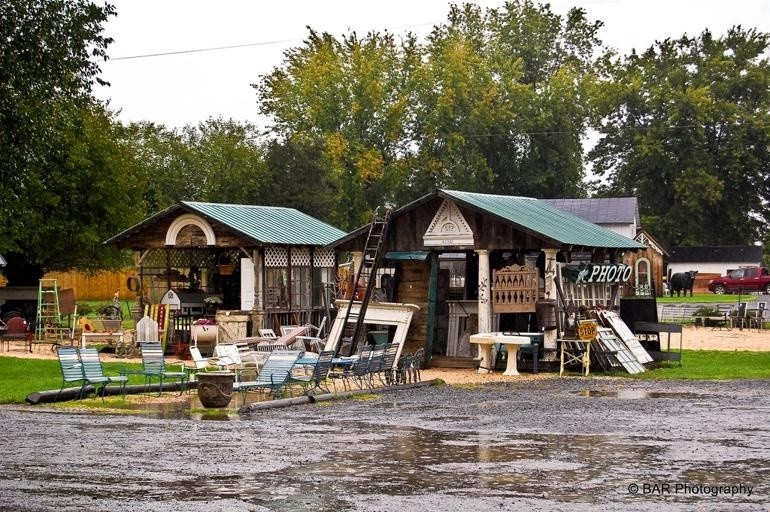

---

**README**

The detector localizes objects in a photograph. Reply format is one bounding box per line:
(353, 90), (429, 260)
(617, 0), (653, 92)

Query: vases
(195, 373), (235, 408)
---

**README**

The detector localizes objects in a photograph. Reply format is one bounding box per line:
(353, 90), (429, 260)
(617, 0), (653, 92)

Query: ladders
(34, 279), (62, 341)
(330, 206), (393, 371)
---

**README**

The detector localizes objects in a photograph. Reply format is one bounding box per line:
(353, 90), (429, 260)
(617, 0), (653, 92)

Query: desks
(118, 369), (158, 401)
(555, 339), (591, 376)
(469, 332), (565, 375)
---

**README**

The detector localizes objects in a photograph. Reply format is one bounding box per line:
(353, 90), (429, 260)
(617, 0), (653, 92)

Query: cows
(670, 271), (698, 297)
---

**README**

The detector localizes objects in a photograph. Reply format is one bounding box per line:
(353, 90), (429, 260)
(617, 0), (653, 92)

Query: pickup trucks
(707, 266), (770, 295)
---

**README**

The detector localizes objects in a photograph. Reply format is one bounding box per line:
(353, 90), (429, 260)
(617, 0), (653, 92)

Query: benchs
(79, 319), (124, 349)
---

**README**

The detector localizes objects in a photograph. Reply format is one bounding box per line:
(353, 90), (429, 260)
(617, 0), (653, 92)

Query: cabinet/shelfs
(631, 320), (683, 366)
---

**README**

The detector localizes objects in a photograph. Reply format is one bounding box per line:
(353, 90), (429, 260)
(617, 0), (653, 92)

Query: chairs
(709, 302), (765, 332)
(232, 342), (425, 405)
(57, 347), (106, 402)
(0, 311), (34, 353)
(141, 342), (186, 398)
(190, 345), (229, 372)
(216, 343), (258, 384)
(77, 349), (128, 401)
